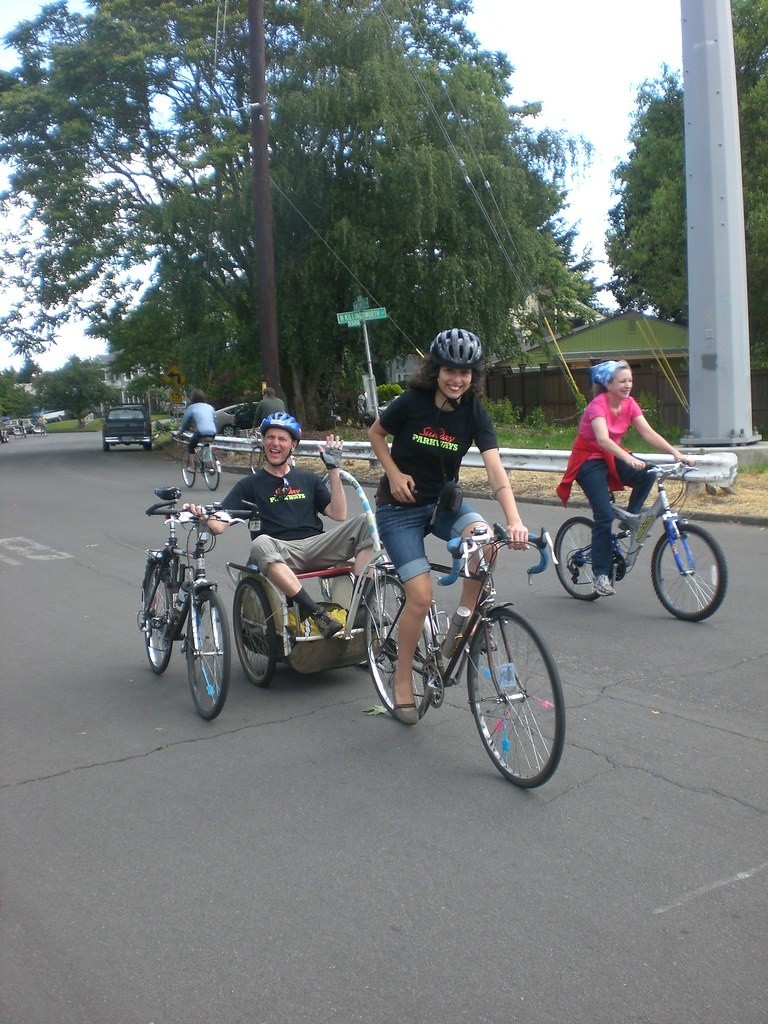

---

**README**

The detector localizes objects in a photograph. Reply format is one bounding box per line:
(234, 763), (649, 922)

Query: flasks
(441, 606), (471, 658)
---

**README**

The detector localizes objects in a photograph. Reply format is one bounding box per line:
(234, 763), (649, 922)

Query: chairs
(112, 414), (120, 419)
(132, 413), (139, 418)
(293, 566), (356, 636)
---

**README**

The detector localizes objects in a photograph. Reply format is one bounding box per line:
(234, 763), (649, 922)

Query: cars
(213, 402), (258, 437)
(41, 409), (65, 423)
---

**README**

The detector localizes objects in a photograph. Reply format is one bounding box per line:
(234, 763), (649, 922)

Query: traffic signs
(336, 298), (388, 327)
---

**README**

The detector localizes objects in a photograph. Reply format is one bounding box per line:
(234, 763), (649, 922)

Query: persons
(367, 328), (528, 725)
(182, 412), (393, 639)
(0, 415), (48, 444)
(178, 388), (216, 474)
(169, 406), (176, 423)
(575, 360), (697, 596)
(249, 387), (286, 435)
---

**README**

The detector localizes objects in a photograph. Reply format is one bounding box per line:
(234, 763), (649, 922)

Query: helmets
(260, 412), (302, 442)
(429, 328), (483, 368)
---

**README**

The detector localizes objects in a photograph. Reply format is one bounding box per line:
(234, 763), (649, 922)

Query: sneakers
(618, 522), (652, 538)
(312, 606), (343, 638)
(355, 606), (391, 628)
(593, 575), (616, 596)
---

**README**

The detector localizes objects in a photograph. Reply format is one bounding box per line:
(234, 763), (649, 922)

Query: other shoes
(468, 632), (499, 651)
(390, 678), (420, 724)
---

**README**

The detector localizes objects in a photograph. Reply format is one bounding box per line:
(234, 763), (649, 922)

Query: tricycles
(12, 425), (26, 438)
(31, 423), (47, 437)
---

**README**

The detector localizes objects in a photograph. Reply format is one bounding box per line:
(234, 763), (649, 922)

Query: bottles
(175, 582), (192, 611)
(166, 580), (180, 615)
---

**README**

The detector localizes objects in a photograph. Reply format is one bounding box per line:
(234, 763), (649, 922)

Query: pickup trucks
(159, 403), (187, 416)
(4, 418), (45, 434)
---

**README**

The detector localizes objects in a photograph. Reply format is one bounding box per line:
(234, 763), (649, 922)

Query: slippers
(185, 467), (195, 473)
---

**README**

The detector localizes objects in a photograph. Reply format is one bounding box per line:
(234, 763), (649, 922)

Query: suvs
(102, 407), (152, 451)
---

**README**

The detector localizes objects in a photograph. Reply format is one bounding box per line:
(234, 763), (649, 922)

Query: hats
(590, 360), (621, 391)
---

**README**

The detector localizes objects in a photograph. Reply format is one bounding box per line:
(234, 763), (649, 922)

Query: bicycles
(364, 523), (566, 788)
(250, 429), (295, 474)
(179, 432), (221, 491)
(552, 461), (729, 621)
(136, 486), (261, 720)
